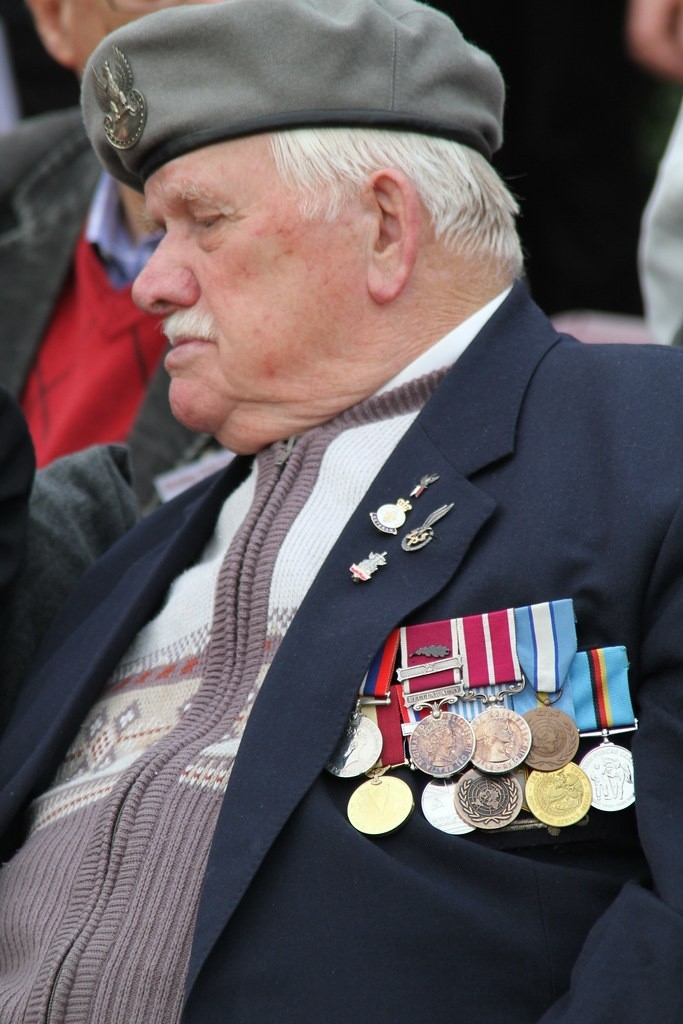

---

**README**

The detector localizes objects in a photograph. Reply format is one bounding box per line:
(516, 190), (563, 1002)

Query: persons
(0, 0), (228, 614)
(0, 0), (682, 1024)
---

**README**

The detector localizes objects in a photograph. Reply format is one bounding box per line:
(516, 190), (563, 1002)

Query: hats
(82, 2), (505, 193)
